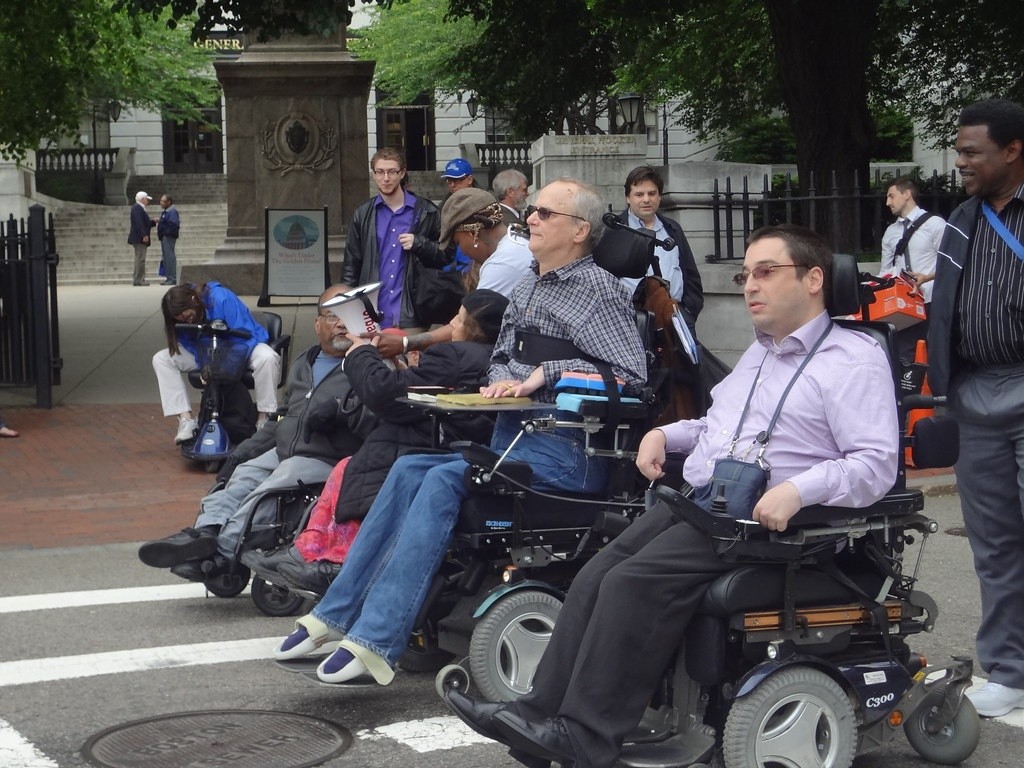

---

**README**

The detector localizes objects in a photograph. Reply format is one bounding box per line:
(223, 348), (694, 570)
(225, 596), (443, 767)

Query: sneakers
(174, 417), (200, 446)
(968, 682), (1024, 716)
(241, 542), (344, 601)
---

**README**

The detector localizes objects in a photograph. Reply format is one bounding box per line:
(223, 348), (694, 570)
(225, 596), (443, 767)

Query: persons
(127, 191), (159, 286)
(156, 194), (180, 286)
(139, 147), (653, 695)
(925, 97), (1024, 715)
(443, 223), (900, 768)
(863, 175), (948, 365)
(590, 165), (705, 422)
(152, 280), (283, 446)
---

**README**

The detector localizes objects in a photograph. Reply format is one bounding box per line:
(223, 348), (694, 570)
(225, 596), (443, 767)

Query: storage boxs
(852, 274), (926, 333)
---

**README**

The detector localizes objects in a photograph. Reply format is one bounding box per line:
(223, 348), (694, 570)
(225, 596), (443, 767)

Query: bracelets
(402, 335), (408, 355)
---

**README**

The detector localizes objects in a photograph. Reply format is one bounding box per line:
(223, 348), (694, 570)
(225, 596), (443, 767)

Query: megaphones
(321, 281), (398, 409)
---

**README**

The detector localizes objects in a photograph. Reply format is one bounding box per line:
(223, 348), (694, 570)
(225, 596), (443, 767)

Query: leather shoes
(443, 689), (577, 767)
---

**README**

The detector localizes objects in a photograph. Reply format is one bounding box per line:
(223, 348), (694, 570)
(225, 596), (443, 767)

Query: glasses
(732, 264), (812, 287)
(522, 186), (528, 193)
(445, 175), (470, 184)
(527, 205), (586, 222)
(375, 169), (402, 176)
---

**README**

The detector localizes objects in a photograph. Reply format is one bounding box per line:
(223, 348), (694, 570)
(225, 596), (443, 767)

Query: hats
(461, 289), (510, 344)
(135, 191), (153, 200)
(438, 187), (497, 251)
(441, 157), (473, 179)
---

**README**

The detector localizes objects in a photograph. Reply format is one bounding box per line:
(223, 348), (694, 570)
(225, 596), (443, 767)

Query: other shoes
(140, 527), (231, 580)
(134, 281), (150, 286)
(160, 279), (176, 285)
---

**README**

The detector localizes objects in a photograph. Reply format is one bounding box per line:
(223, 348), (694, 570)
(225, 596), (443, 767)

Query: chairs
(189, 311), (291, 389)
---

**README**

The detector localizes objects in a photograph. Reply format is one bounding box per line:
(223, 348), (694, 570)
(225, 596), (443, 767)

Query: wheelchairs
(201, 219), (706, 672)
(175, 309), (291, 473)
(615, 253), (988, 764)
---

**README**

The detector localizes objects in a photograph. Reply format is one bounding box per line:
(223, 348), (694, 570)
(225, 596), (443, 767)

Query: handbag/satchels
(414, 265), (466, 325)
(159, 261), (166, 276)
(693, 461), (768, 521)
